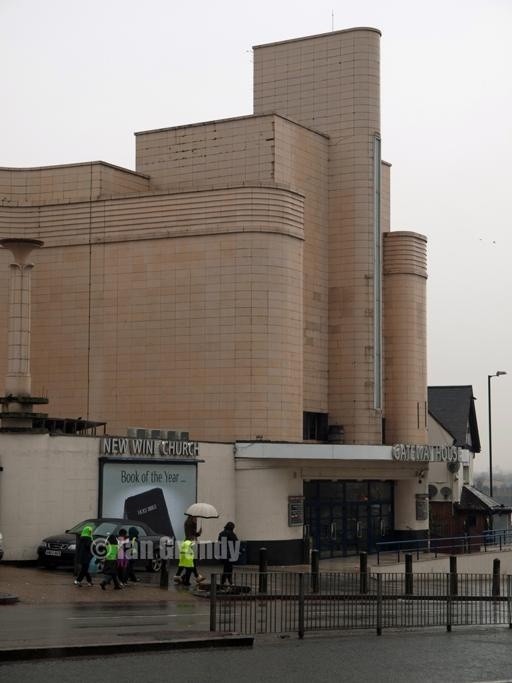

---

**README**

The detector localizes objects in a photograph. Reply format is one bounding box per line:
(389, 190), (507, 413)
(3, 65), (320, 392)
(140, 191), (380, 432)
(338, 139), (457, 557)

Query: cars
(37, 517), (172, 572)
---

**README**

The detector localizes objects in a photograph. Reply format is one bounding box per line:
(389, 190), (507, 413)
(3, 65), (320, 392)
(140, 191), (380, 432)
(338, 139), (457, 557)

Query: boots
(195, 576), (207, 583)
(174, 576), (182, 583)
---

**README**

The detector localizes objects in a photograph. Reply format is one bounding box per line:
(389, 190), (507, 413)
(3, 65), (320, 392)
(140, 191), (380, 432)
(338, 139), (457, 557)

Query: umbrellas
(184, 503), (219, 528)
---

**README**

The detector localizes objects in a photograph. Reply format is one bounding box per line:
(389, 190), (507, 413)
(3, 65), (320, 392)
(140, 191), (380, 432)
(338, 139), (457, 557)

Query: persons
(218, 522), (239, 585)
(99, 526), (140, 590)
(178, 536), (195, 586)
(174, 516), (206, 583)
(75, 526), (96, 587)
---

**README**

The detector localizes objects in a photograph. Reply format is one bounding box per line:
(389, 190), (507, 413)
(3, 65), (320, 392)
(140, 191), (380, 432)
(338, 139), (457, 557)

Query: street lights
(488, 370), (506, 544)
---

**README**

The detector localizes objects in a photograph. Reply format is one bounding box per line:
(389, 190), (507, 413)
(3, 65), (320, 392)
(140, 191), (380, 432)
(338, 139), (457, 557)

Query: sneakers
(100, 579), (140, 589)
(181, 575), (191, 586)
(74, 580), (95, 587)
